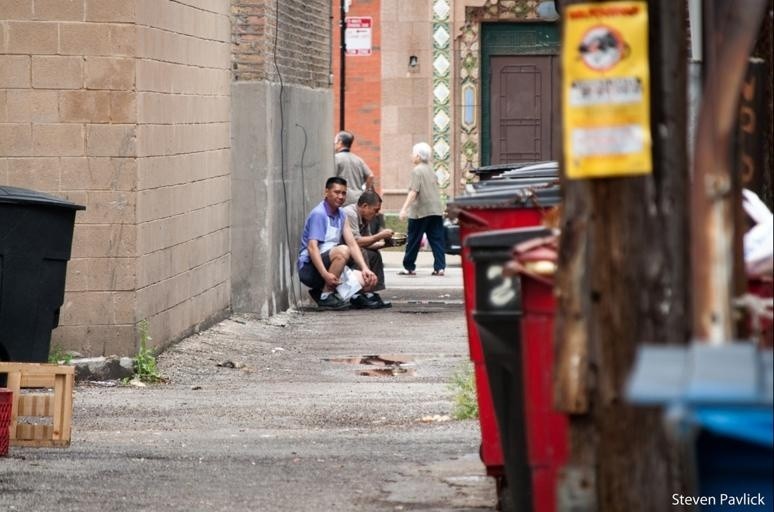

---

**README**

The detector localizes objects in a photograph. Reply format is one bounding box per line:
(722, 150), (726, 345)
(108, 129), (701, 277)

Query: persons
(333, 130), (376, 209)
(340, 189), (394, 309)
(296, 176), (380, 311)
(396, 142), (447, 276)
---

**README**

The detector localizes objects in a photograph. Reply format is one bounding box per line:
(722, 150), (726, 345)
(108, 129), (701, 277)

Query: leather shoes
(309, 288), (392, 310)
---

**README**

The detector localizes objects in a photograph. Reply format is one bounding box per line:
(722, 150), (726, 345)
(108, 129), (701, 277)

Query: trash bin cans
(0, 186), (86, 364)
(447, 160), (562, 512)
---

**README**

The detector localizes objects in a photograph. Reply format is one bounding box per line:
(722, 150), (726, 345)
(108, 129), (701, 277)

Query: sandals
(432, 269), (445, 276)
(398, 269), (416, 275)
